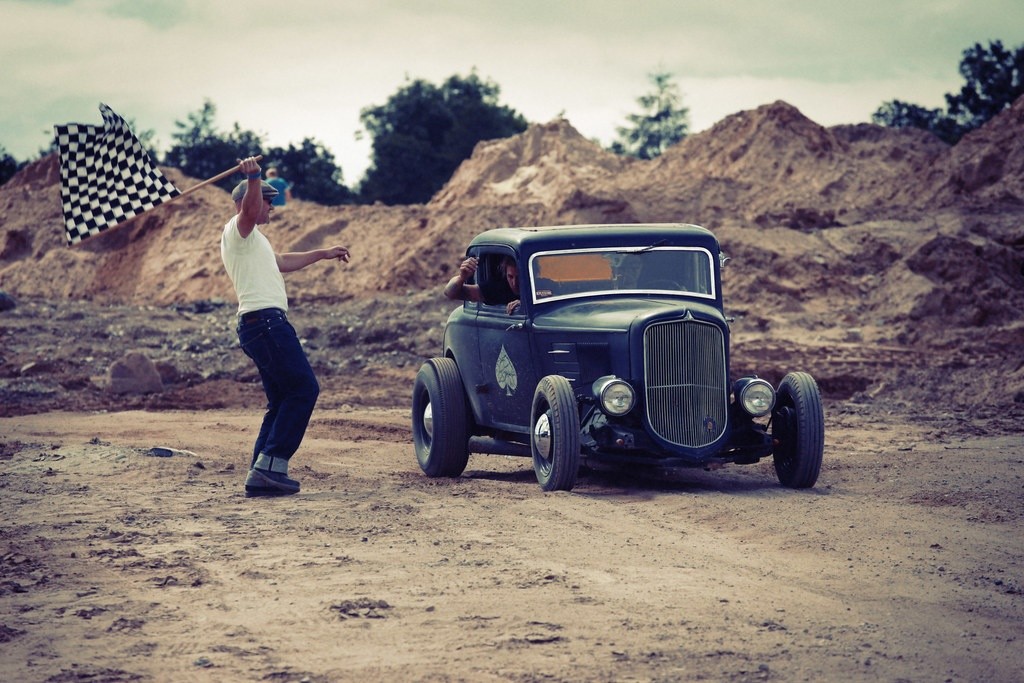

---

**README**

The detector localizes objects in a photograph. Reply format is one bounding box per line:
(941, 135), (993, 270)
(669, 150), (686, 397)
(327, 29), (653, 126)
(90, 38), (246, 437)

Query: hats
(232, 179), (279, 203)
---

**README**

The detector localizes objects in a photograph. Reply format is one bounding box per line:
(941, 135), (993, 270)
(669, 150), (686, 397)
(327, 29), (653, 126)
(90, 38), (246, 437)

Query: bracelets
(247, 173), (261, 179)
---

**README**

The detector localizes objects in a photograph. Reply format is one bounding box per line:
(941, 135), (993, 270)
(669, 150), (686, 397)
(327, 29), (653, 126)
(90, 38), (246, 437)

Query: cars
(412, 224), (824, 492)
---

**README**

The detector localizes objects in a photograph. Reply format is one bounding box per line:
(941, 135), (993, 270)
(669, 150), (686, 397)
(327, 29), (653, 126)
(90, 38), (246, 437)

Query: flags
(52, 103), (180, 245)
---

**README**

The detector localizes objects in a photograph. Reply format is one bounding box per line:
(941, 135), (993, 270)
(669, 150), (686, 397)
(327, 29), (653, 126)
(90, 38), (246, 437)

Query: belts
(242, 308), (285, 321)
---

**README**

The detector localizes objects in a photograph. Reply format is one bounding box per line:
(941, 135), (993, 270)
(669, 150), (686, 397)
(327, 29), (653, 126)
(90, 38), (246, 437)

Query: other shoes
(250, 466), (300, 493)
(245, 484), (280, 498)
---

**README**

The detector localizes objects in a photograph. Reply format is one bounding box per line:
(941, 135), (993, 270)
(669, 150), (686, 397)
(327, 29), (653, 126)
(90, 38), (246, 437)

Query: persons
(444, 254), (565, 316)
(221, 157), (351, 497)
(263, 168), (292, 206)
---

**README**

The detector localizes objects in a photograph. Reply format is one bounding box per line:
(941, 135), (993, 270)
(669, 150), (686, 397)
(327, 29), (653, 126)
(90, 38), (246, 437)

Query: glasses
(263, 197), (273, 205)
